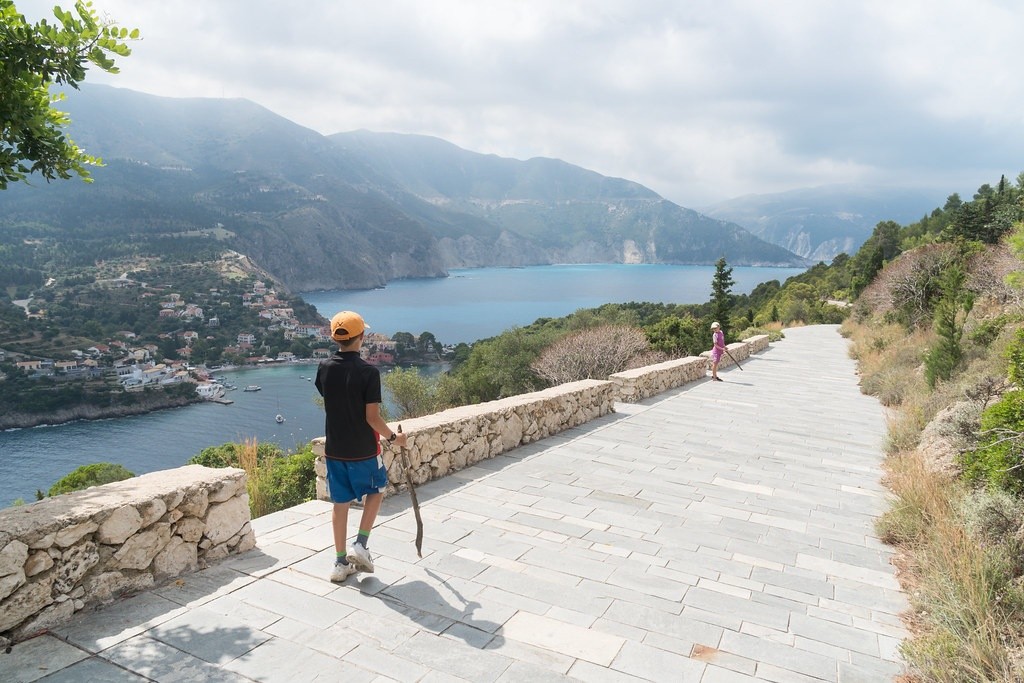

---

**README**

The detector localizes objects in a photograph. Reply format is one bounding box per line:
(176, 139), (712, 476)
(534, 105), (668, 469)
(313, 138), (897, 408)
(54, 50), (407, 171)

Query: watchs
(386, 432), (396, 443)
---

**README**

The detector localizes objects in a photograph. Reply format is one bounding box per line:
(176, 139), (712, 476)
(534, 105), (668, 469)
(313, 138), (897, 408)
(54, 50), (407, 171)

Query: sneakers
(330, 560), (362, 582)
(345, 542), (374, 573)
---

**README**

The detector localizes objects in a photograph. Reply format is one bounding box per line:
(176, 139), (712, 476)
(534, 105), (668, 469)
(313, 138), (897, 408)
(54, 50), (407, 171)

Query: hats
(711, 322), (719, 329)
(330, 311), (371, 341)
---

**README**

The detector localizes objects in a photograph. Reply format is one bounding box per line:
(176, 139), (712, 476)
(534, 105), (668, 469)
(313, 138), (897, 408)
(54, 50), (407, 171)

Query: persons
(710, 322), (728, 381)
(315, 311), (408, 582)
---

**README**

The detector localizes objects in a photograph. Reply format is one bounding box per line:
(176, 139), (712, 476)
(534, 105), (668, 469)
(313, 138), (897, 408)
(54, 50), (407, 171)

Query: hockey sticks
(396, 423), (423, 553)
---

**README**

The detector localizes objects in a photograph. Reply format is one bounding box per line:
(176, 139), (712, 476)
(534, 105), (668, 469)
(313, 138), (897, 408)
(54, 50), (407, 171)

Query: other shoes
(712, 376), (723, 381)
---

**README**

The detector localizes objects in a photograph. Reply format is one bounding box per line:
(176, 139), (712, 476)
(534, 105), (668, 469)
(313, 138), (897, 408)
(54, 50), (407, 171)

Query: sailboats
(275, 392), (284, 423)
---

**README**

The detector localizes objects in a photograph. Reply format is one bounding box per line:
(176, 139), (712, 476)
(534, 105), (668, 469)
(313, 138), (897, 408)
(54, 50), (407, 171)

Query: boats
(243, 384), (262, 392)
